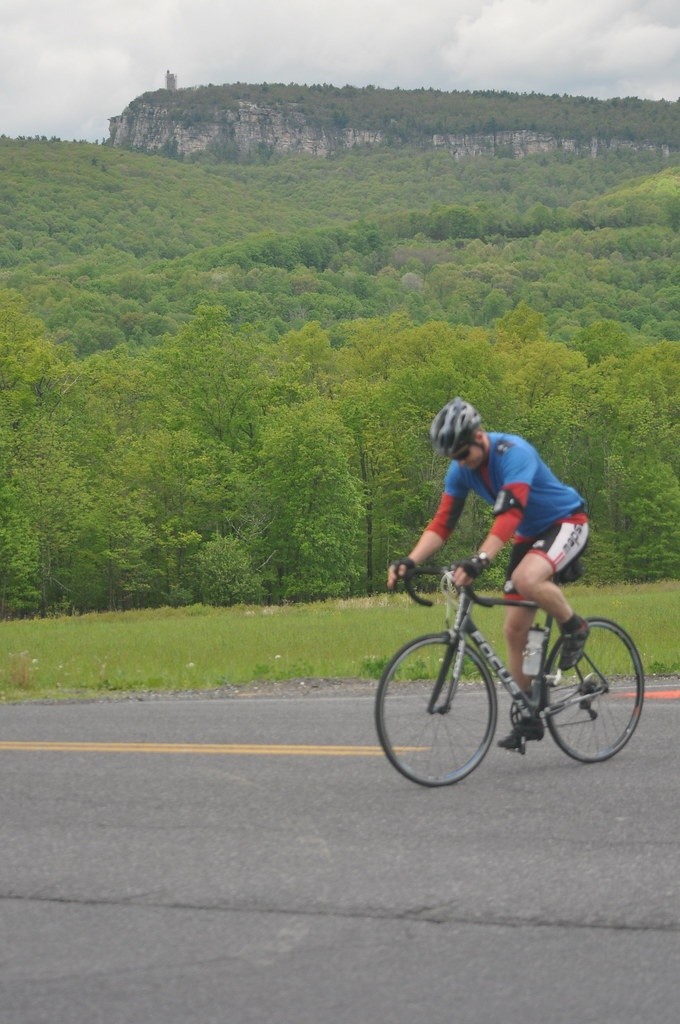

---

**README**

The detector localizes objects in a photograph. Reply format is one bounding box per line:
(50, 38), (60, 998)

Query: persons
(387, 396), (591, 753)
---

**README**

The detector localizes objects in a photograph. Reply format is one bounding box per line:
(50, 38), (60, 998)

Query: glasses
(452, 445), (470, 460)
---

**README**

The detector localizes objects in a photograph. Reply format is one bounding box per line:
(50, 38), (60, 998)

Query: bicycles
(373, 556), (647, 789)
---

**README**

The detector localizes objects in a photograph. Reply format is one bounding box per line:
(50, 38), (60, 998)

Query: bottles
(522, 624), (544, 675)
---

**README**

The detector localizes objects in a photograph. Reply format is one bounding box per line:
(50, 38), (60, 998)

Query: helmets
(430, 397), (483, 457)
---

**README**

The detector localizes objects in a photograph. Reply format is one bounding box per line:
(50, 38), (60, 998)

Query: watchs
(476, 550), (490, 564)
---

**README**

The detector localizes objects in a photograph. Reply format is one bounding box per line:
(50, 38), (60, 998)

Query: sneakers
(497, 717), (544, 748)
(557, 622), (589, 670)
